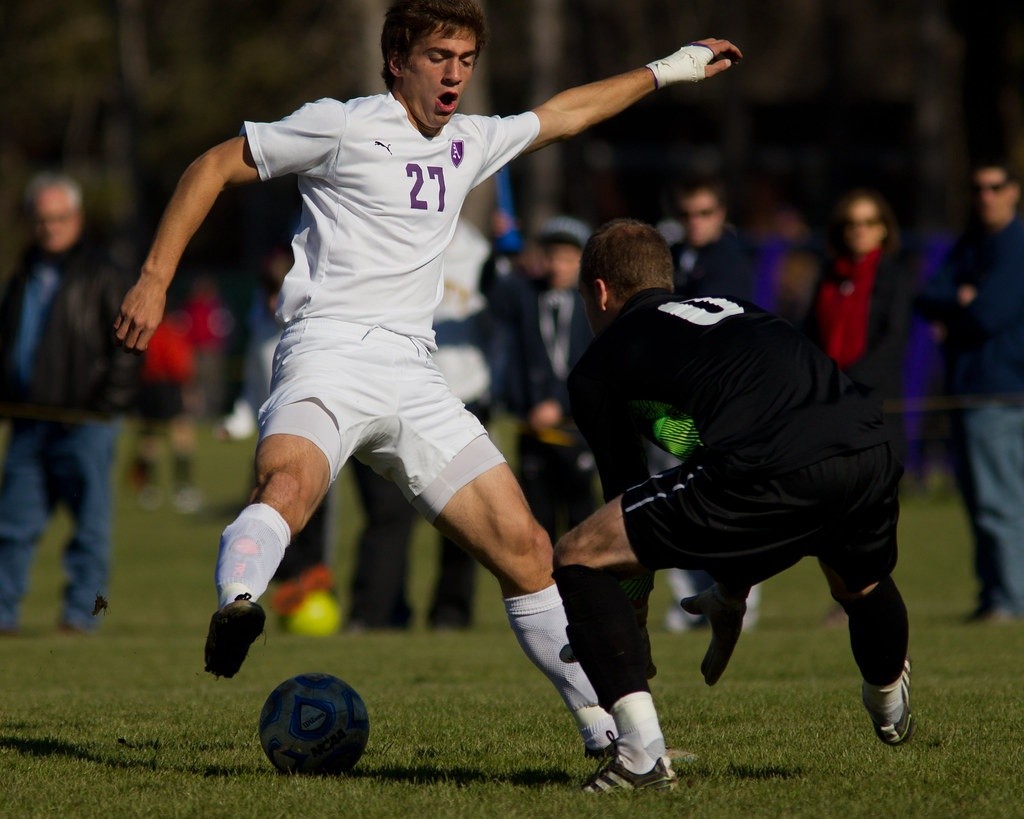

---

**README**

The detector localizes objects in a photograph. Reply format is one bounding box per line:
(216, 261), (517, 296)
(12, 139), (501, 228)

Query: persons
(1, 183), (148, 637)
(925, 160), (1023, 623)
(122, 185), (904, 633)
(550, 220), (914, 794)
(113, 1), (743, 760)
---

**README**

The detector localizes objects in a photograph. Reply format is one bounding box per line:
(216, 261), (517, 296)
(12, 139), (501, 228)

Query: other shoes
(968, 604), (1013, 625)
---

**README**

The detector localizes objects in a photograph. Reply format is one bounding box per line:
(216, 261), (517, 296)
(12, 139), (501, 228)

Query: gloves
(681, 579), (747, 687)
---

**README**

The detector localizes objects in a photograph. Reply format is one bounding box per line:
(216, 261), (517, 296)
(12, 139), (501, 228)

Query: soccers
(257, 670), (373, 779)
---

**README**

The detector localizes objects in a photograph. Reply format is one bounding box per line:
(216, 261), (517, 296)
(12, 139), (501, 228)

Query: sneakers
(581, 732), (677, 795)
(585, 737), (619, 757)
(203, 593), (265, 679)
(860, 656), (911, 745)
